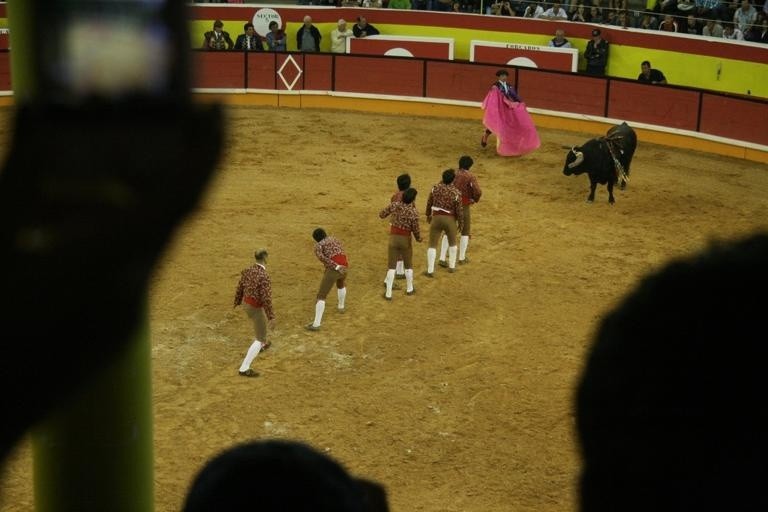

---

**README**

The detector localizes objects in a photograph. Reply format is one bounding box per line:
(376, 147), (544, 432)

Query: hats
(495, 70), (507, 76)
(592, 29), (600, 36)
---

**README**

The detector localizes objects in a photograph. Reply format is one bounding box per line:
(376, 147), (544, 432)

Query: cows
(562, 122), (638, 206)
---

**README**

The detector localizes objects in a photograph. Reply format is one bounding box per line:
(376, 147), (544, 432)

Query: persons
(182, 438), (389, 509)
(549, 28), (571, 49)
(638, 61), (666, 84)
(233, 249), (277, 379)
(422, 168), (465, 277)
(352, 15), (380, 38)
(566, 234), (767, 512)
(204, 19), (234, 50)
(438, 156), (482, 267)
(265, 21), (287, 51)
(296, 15), (322, 52)
(331, 18), (354, 53)
(584, 28), (609, 78)
(303, 228), (349, 332)
(234, 22), (264, 51)
(378, 188), (423, 301)
(382, 174), (406, 290)
(1, 84), (225, 472)
(338, 3), (766, 43)
(480, 69), (525, 147)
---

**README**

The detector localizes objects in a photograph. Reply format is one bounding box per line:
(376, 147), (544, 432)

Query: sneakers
(338, 305), (348, 313)
(383, 257), (467, 304)
(260, 341), (270, 352)
(304, 324), (319, 330)
(239, 368), (259, 377)
